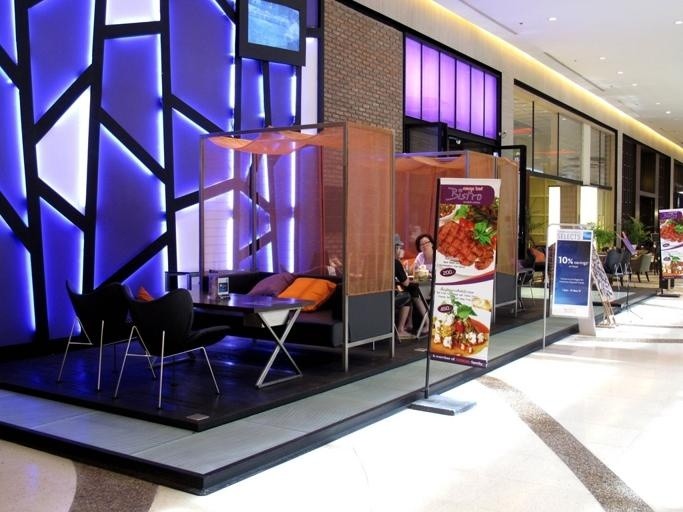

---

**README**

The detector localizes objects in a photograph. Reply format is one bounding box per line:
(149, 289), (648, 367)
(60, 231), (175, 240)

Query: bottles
(208, 269), (230, 300)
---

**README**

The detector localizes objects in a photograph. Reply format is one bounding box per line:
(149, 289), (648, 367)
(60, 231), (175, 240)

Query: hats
(395, 233), (404, 246)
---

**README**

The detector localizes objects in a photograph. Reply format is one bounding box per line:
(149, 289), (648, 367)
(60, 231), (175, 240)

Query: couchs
(165, 270), (342, 372)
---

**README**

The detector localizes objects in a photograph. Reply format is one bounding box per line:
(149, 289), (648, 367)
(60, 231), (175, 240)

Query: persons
(395, 233), (417, 339)
(644, 234), (653, 250)
(410, 234), (434, 333)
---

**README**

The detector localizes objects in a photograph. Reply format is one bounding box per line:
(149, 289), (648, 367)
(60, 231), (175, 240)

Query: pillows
(247, 271), (339, 314)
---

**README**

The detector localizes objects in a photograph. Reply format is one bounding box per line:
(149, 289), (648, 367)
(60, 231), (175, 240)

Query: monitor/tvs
(236, 0), (307, 67)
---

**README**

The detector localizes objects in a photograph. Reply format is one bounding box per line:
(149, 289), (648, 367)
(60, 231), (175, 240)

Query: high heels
(420, 329), (429, 336)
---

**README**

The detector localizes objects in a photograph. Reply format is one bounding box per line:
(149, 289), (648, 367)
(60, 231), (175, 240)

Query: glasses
(420, 241), (430, 246)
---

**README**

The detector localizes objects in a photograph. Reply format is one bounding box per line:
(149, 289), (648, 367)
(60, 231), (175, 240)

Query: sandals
(398, 332), (416, 339)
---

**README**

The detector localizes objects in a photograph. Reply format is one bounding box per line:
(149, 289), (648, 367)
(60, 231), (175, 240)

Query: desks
(192, 291), (316, 390)
(394, 274), (434, 343)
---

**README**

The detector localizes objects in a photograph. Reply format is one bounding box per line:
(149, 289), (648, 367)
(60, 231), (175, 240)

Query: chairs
(600, 248), (652, 290)
(57, 280), (231, 410)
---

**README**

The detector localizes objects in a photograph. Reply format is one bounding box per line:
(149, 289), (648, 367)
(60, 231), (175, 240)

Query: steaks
(437, 221), (496, 270)
(660, 223), (683, 242)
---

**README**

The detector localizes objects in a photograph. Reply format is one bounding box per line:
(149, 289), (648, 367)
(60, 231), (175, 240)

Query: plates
(430, 318), (490, 356)
